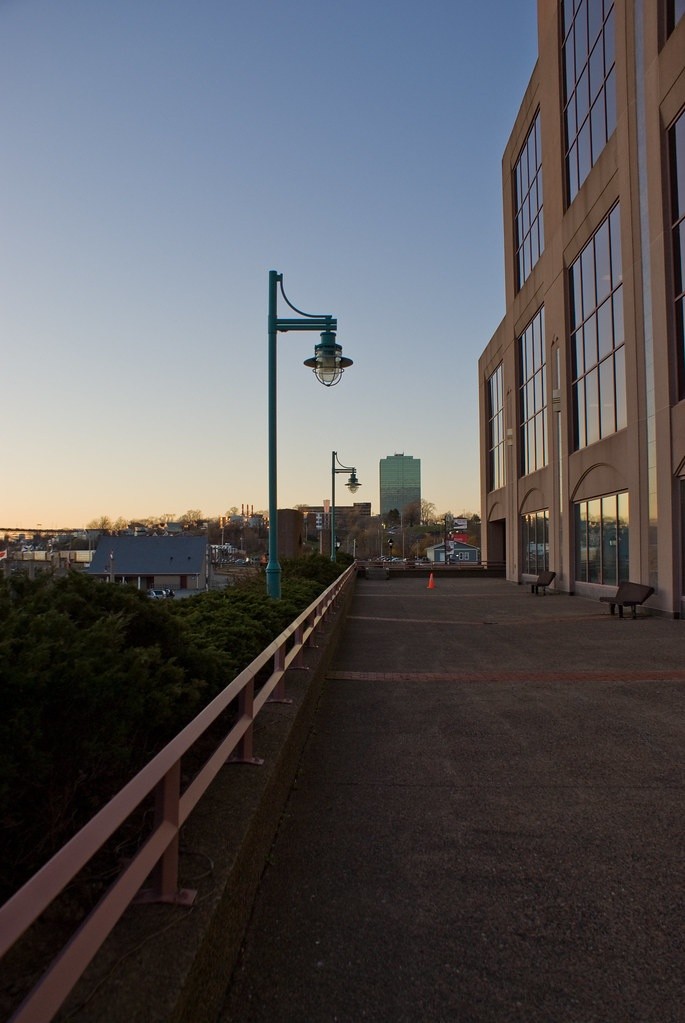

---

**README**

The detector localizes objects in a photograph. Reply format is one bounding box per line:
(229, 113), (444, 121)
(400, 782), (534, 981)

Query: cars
(144, 589), (175, 601)
(373, 555), (430, 569)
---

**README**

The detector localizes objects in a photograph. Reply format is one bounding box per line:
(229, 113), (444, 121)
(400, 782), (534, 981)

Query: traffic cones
(426, 572), (436, 589)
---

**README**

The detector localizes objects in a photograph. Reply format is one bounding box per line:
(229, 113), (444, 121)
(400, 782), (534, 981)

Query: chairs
(525, 570), (557, 596)
(596, 580), (656, 622)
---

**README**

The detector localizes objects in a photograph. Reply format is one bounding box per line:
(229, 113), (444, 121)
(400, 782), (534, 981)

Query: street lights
(266, 271), (356, 599)
(329, 450), (363, 563)
(387, 539), (394, 564)
(440, 516), (451, 566)
